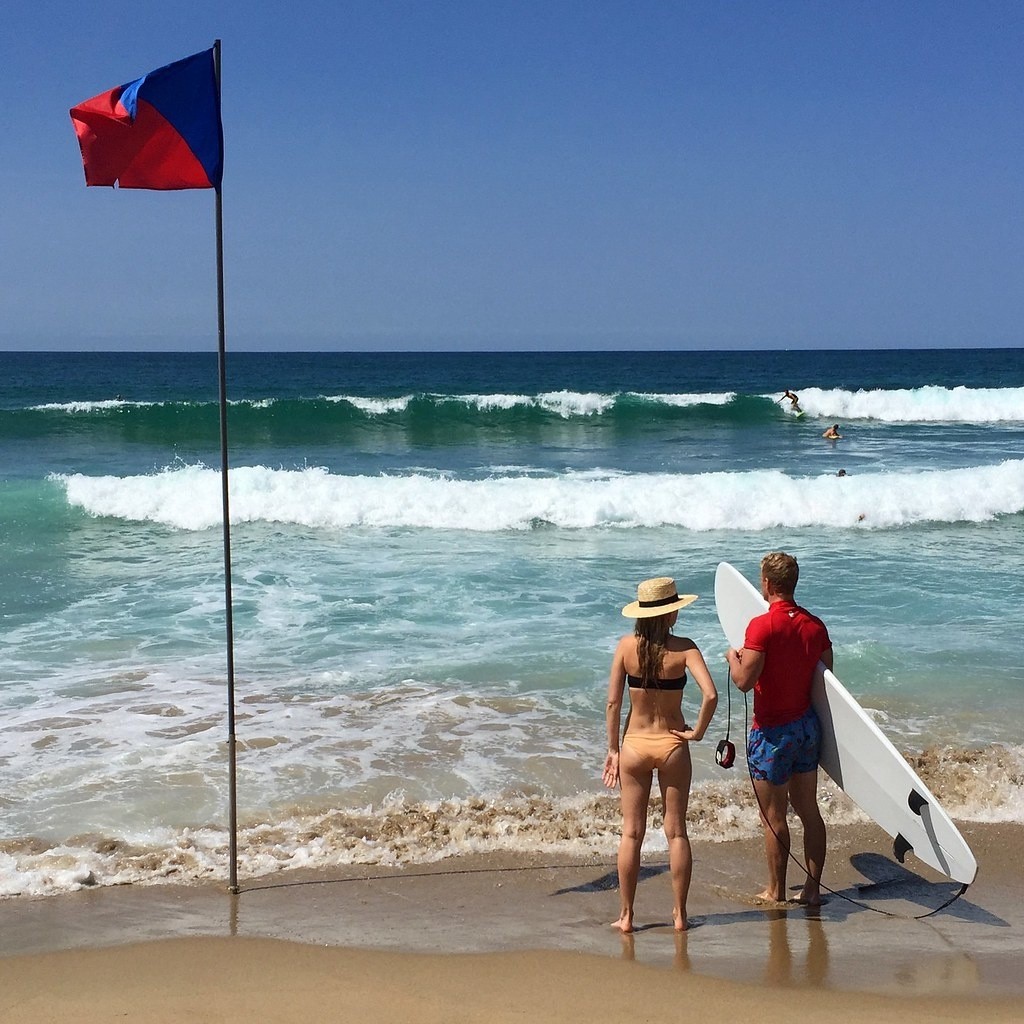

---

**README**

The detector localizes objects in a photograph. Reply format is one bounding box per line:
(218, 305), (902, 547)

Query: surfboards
(714, 557), (979, 884)
(794, 407), (805, 417)
(830, 435), (841, 439)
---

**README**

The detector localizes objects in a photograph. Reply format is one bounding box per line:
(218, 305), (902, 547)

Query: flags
(70, 47), (222, 191)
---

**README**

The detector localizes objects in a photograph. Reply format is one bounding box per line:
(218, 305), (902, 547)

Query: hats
(621, 577), (698, 619)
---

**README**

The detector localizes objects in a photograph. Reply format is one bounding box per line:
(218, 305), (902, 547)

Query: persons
(824, 424), (839, 436)
(778, 390), (799, 406)
(602, 577), (718, 934)
(838, 469), (846, 476)
(727, 551), (834, 905)
